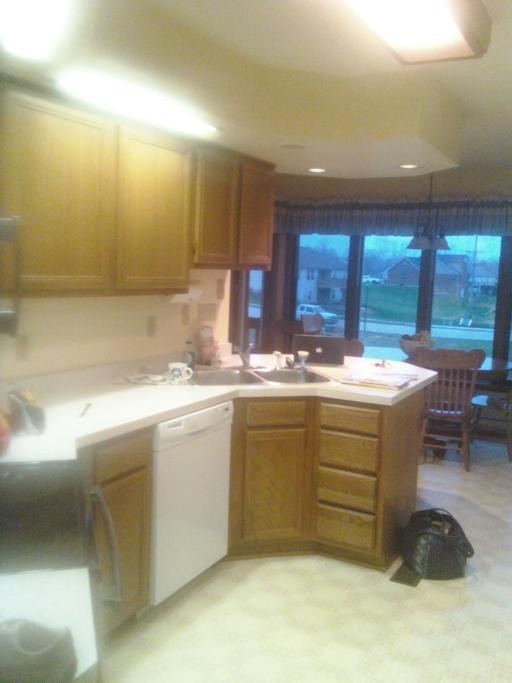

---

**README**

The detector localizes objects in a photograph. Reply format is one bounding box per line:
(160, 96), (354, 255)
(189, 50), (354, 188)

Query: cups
(168, 362), (193, 385)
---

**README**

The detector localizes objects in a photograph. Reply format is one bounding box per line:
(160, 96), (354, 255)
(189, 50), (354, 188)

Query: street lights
(364, 281), (370, 331)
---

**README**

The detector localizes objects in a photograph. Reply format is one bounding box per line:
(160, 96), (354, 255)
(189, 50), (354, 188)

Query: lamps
(406, 173), (451, 251)
(341, 0), (491, 67)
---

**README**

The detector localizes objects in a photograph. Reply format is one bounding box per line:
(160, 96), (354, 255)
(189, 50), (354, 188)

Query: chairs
(343, 339), (364, 358)
(457, 388), (512, 463)
(411, 345), (485, 470)
(278, 313), (324, 355)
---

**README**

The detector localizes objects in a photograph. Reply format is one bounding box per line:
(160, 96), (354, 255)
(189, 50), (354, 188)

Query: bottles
(182, 339), (196, 372)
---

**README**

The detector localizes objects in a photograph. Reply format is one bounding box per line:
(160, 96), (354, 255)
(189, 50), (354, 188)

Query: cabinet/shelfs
(0, 84), (196, 297)
(192, 139), (276, 271)
(312, 393), (423, 571)
(89, 432), (152, 641)
(243, 400), (308, 555)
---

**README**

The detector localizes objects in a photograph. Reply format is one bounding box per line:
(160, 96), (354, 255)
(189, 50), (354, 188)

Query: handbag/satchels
(401, 507), (475, 580)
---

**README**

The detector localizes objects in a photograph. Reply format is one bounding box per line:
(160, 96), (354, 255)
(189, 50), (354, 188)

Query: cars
(296, 304), (337, 324)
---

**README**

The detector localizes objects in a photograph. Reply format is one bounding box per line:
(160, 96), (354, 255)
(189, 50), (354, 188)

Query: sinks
(252, 364), (333, 385)
(188, 364), (265, 388)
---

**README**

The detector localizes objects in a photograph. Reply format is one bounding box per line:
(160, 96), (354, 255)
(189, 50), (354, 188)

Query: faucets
(287, 355), (299, 370)
(232, 342), (256, 368)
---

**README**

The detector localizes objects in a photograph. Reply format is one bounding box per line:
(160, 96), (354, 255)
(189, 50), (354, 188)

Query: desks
(402, 356), (512, 455)
